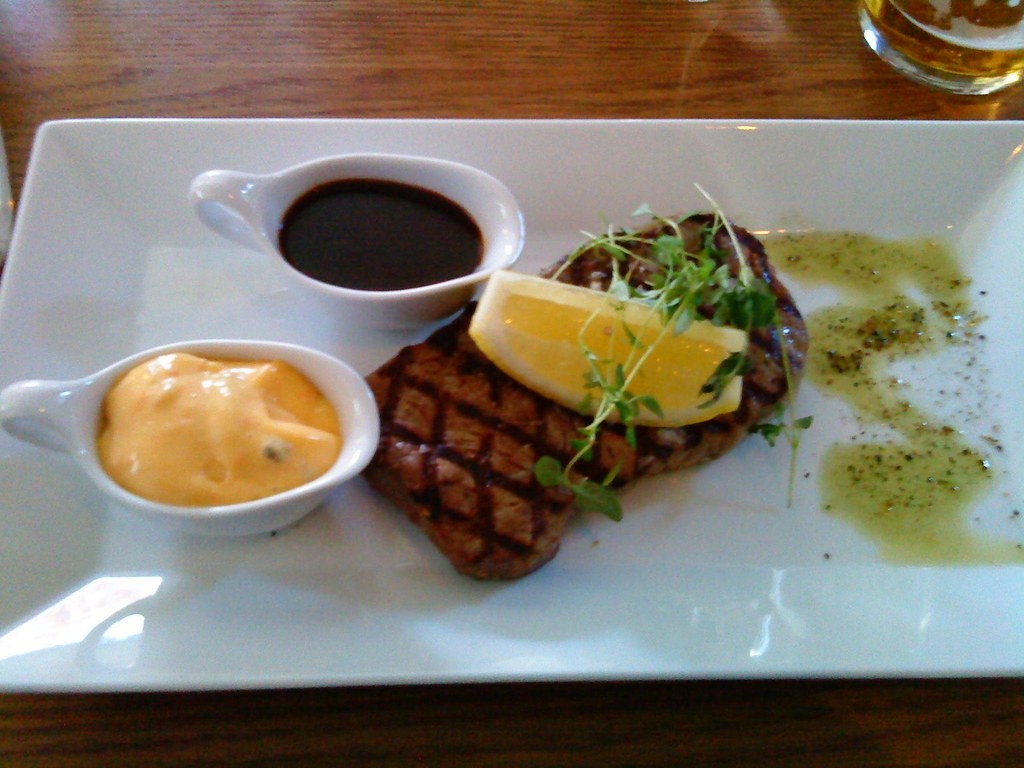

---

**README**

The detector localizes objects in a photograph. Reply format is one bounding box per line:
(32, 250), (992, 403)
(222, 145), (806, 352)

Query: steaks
(358, 216), (806, 581)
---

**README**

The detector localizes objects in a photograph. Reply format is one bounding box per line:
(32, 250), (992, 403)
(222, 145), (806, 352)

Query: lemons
(469, 273), (748, 427)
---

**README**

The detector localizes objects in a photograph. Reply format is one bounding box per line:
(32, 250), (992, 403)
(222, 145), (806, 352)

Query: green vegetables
(536, 184), (813, 523)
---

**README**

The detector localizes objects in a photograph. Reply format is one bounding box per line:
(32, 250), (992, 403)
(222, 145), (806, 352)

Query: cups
(191, 154), (526, 328)
(0, 340), (380, 537)
(859, 0), (1024, 95)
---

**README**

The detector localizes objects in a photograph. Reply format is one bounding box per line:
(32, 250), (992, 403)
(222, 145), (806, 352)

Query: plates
(0, 117), (1024, 692)
